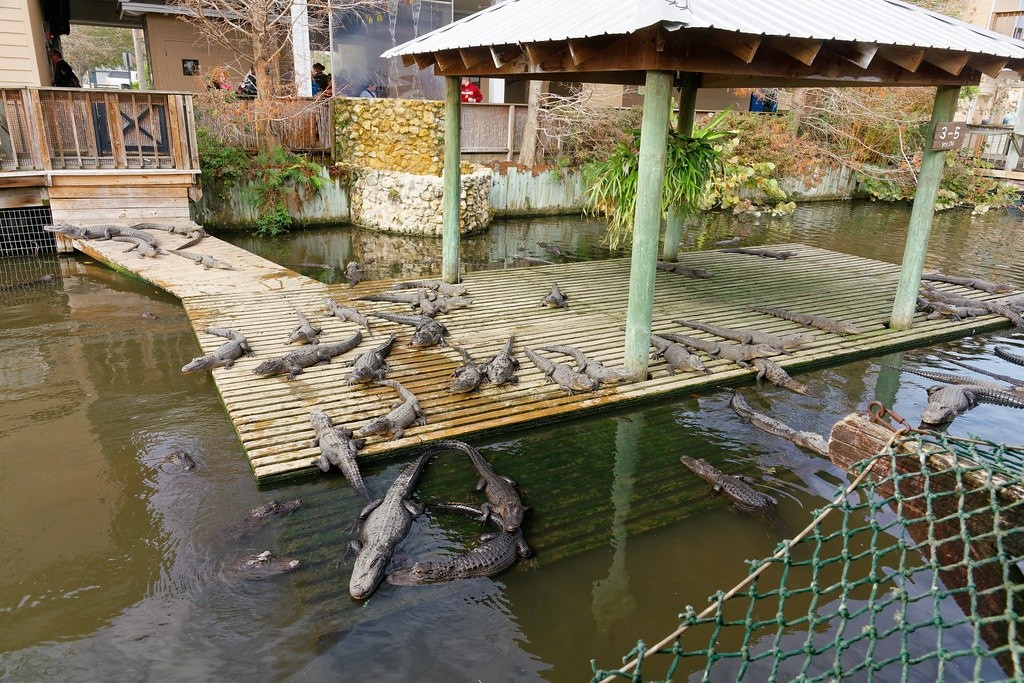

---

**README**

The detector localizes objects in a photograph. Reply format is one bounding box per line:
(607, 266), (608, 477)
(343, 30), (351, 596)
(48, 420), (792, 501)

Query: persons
(460, 76), (482, 103)
(749, 88), (778, 116)
(49, 49), (81, 87)
(235, 65), (295, 100)
(206, 69), (232, 93)
(311, 63), (377, 97)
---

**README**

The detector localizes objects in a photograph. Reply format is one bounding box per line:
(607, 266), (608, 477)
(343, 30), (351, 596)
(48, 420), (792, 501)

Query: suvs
(105, 70), (138, 91)
